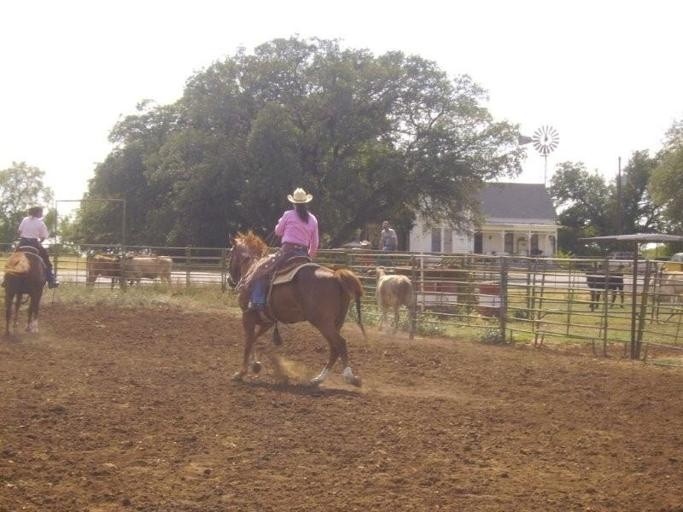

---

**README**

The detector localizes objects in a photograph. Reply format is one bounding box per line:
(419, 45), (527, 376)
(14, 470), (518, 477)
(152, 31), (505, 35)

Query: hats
(287, 188), (313, 204)
(24, 202), (45, 210)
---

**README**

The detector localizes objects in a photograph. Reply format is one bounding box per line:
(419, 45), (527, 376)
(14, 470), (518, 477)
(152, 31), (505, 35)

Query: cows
(86, 248), (174, 292)
(366, 266), (416, 340)
(581, 265), (624, 312)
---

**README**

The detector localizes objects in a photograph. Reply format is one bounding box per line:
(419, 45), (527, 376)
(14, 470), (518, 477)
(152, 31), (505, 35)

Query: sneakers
(244, 304), (263, 314)
(1, 280), (8, 287)
(49, 281), (60, 289)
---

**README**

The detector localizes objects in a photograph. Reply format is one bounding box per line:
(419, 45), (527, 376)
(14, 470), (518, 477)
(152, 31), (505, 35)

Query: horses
(2, 251), (47, 336)
(227, 229), (366, 388)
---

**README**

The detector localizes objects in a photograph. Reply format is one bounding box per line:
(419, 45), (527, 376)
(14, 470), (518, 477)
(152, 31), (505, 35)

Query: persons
(1, 204), (59, 289)
(243, 184), (320, 313)
(378, 219), (400, 250)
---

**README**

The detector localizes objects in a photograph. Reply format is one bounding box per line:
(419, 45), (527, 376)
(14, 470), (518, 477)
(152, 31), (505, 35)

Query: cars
(602, 251), (683, 277)
